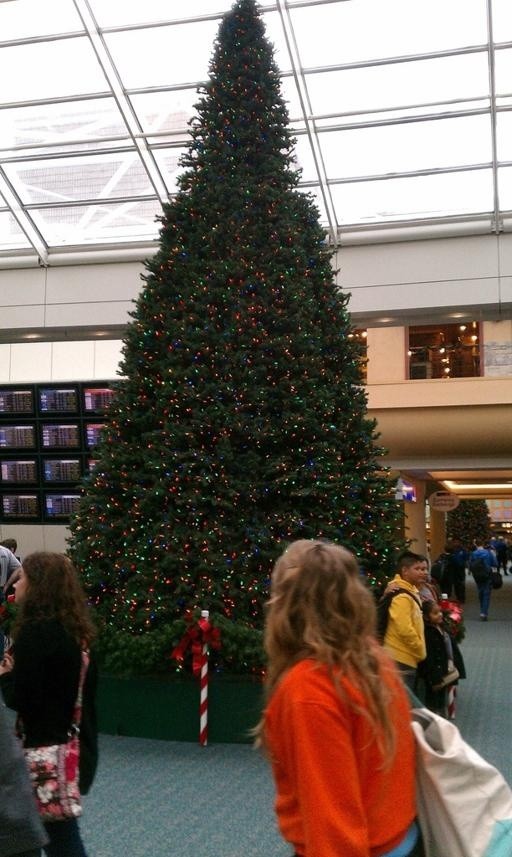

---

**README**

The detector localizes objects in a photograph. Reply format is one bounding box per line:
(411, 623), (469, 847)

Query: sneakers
(480, 613), (488, 621)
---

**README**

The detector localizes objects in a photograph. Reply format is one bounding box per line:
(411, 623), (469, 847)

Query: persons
(0, 539), (98, 857)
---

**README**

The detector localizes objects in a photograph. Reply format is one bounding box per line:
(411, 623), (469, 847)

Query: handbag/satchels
(492, 572), (502, 589)
(431, 563), (445, 581)
(403, 684), (512, 857)
(23, 735), (84, 822)
(375, 588), (422, 646)
